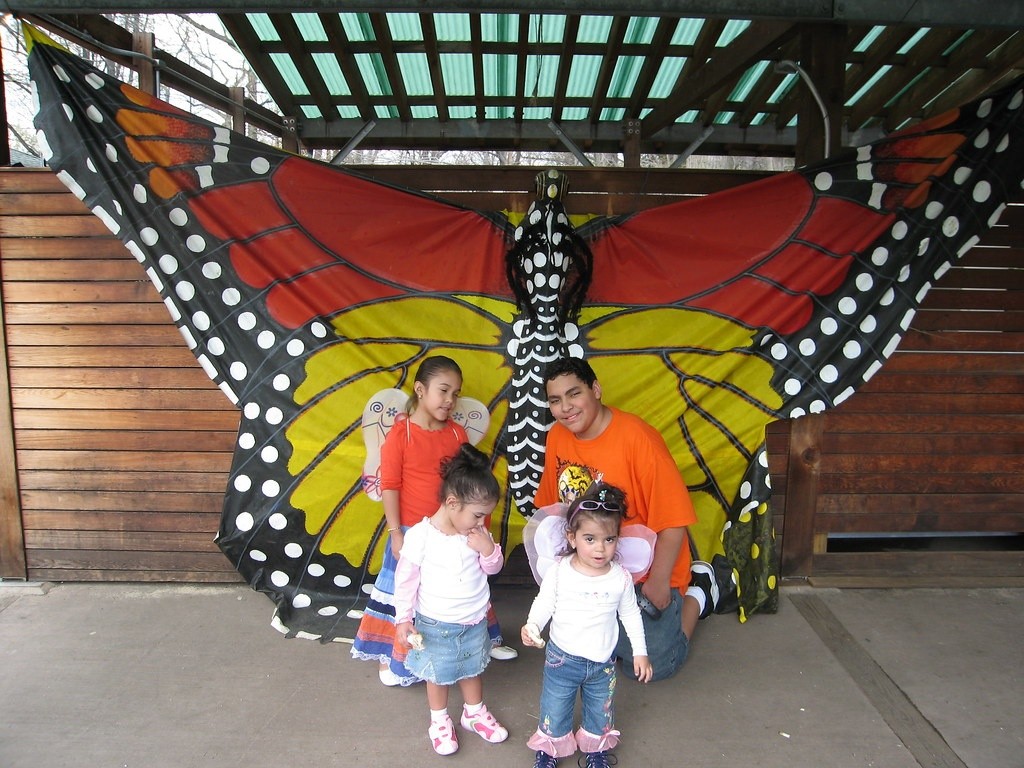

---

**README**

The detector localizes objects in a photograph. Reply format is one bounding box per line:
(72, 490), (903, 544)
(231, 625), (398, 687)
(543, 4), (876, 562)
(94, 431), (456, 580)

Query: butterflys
(9, 12), (1024, 646)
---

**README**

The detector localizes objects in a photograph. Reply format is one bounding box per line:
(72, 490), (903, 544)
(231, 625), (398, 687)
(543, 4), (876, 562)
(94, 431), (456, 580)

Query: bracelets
(388, 526), (401, 532)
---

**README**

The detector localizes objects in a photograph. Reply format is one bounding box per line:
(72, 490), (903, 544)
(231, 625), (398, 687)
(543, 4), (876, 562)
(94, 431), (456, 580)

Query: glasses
(569, 500), (622, 529)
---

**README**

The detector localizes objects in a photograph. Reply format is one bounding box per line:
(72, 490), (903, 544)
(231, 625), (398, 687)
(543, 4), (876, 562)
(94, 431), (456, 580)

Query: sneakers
(689, 561), (720, 620)
(532, 750), (558, 768)
(578, 752), (618, 768)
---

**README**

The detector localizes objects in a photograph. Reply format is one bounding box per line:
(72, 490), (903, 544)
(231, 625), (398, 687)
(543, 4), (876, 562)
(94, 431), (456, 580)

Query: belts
(636, 592), (662, 621)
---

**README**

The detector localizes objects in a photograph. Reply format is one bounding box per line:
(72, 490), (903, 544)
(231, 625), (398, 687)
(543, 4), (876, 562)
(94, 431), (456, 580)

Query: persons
(351, 354), (518, 686)
(521, 484), (653, 768)
(394, 442), (509, 756)
(535, 357), (720, 681)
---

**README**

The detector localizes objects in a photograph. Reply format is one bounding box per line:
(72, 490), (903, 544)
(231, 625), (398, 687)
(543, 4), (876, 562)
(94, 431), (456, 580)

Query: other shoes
(490, 644), (517, 661)
(379, 669), (401, 687)
(460, 705), (508, 743)
(429, 715), (459, 756)
